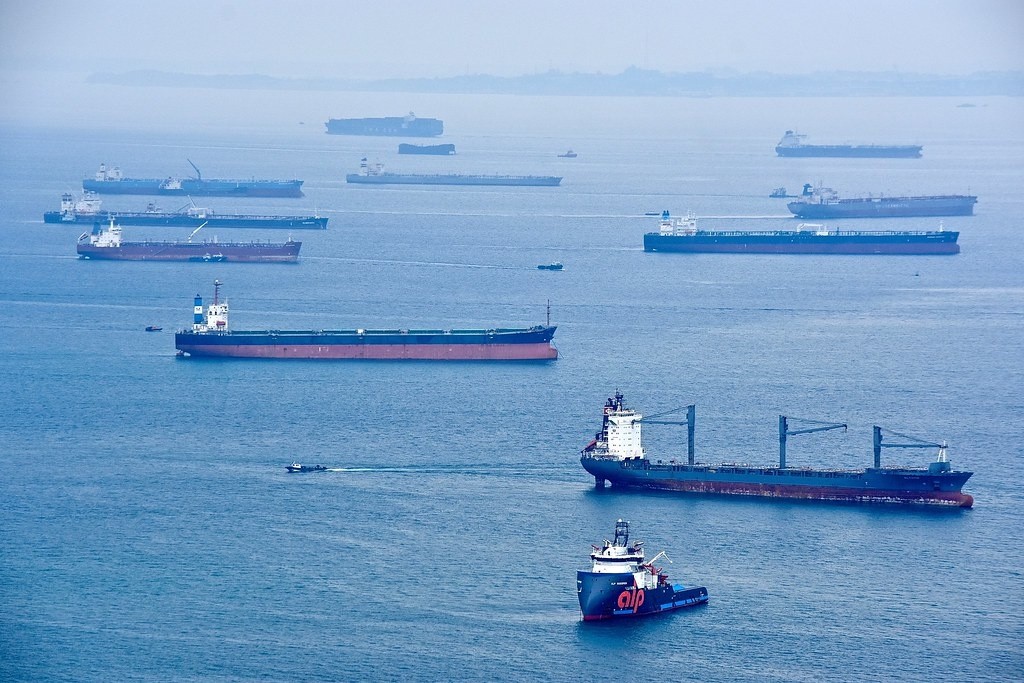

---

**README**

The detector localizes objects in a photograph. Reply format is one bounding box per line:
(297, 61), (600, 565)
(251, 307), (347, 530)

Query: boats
(557, 150), (578, 158)
(645, 213), (660, 216)
(397, 142), (456, 155)
(576, 518), (709, 623)
(538, 261), (563, 271)
(344, 156), (563, 187)
(643, 212), (961, 256)
(76, 216), (303, 264)
(324, 116), (445, 137)
(174, 279), (560, 362)
(42, 189), (329, 230)
(578, 387), (975, 509)
(285, 461), (327, 473)
(787, 182), (979, 220)
(769, 188), (798, 199)
(145, 325), (163, 332)
(82, 157), (305, 199)
(774, 129), (924, 160)
